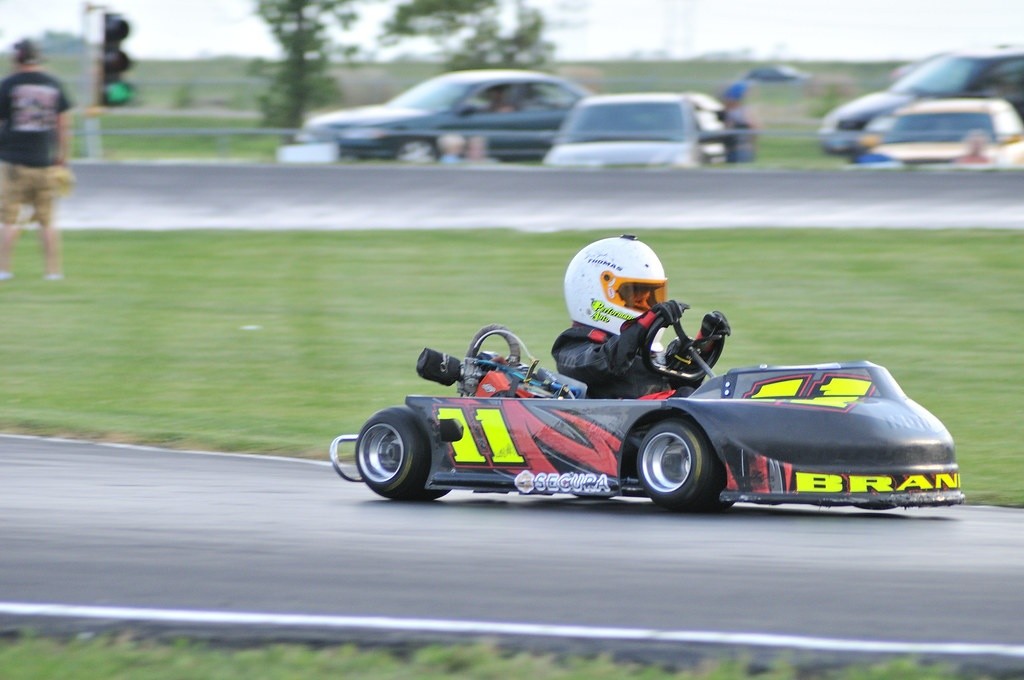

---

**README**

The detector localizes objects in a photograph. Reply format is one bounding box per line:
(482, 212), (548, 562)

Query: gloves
(694, 310), (731, 353)
(636, 299), (690, 335)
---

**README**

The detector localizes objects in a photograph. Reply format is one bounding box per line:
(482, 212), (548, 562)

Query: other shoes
(46, 274), (63, 281)
(0, 271), (13, 279)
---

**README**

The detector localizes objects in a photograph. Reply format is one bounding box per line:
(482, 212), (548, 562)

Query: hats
(14, 40), (38, 62)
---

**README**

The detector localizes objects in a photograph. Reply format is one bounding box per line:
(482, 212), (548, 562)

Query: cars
(815, 49), (1024, 155)
(544, 89), (738, 168)
(294, 64), (596, 163)
(854, 96), (1024, 169)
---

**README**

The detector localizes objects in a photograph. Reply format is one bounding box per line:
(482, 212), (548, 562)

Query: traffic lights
(100, 16), (135, 112)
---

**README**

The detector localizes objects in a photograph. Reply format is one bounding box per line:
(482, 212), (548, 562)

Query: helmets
(563, 234), (668, 336)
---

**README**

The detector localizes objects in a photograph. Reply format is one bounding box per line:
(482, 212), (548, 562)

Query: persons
(551, 235), (729, 398)
(0, 36), (77, 281)
